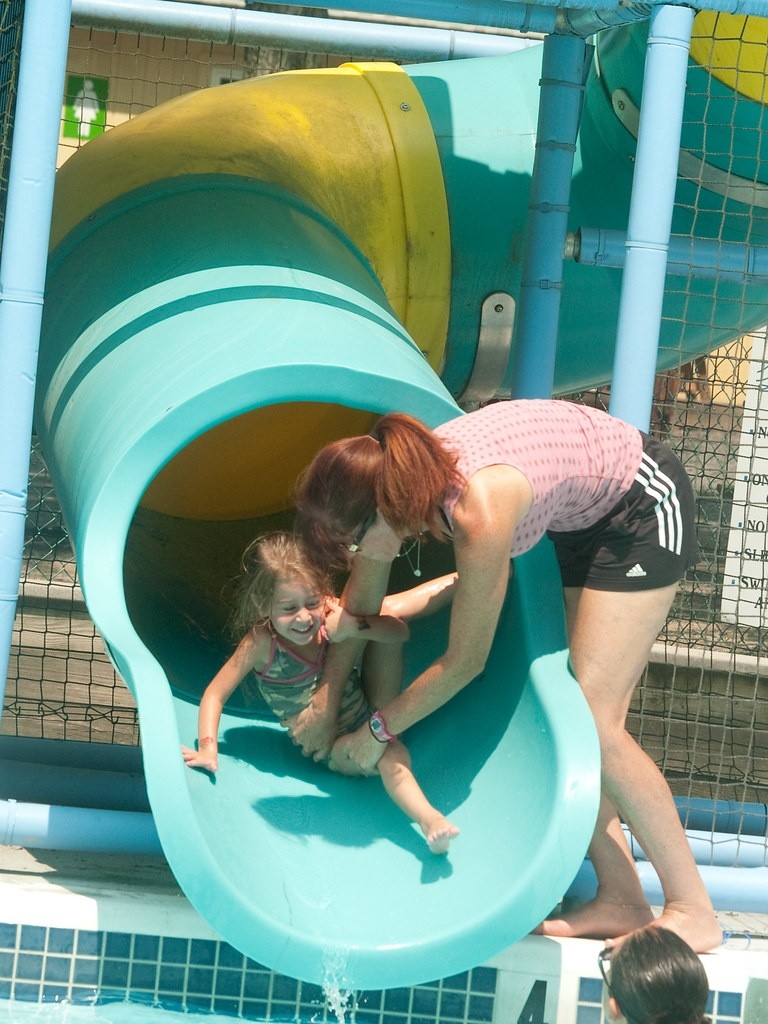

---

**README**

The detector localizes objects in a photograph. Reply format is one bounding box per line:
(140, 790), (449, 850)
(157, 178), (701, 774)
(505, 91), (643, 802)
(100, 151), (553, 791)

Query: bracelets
(369, 718), (391, 743)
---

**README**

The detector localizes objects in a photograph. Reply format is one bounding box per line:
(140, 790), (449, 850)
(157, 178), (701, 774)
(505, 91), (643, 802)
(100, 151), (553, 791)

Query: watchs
(370, 711), (397, 744)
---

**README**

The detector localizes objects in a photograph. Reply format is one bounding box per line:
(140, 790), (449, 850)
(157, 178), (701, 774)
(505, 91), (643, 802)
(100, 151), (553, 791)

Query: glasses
(339, 509), (378, 553)
(598, 946), (616, 992)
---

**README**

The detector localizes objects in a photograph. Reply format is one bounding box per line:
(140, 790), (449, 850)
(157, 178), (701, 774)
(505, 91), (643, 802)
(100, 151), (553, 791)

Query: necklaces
(396, 530), (424, 578)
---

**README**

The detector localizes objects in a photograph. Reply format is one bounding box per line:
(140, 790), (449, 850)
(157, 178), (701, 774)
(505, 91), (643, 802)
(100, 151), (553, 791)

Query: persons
(181, 532), (464, 857)
(601, 925), (709, 1024)
(289, 398), (726, 958)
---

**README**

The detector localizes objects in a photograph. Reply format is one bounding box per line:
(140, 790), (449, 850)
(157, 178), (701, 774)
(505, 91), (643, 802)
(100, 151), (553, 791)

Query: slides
(34, 6), (768, 992)
